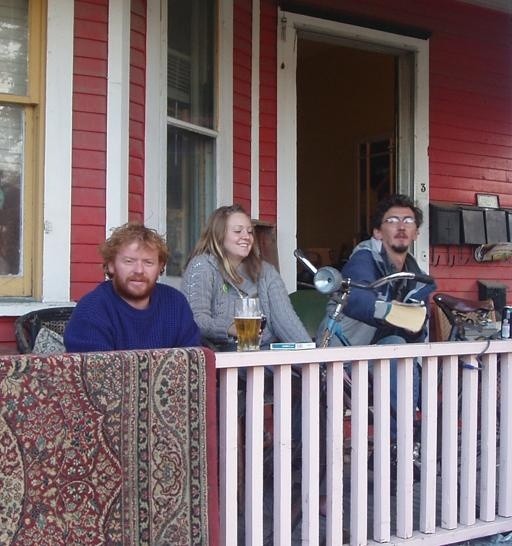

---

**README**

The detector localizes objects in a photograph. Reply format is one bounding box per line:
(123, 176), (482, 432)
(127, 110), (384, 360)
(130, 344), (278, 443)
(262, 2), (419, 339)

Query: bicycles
(215, 249), (499, 544)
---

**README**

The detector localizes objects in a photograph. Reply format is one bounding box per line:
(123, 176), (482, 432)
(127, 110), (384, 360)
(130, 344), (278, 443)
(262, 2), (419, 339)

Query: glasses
(383, 215), (416, 224)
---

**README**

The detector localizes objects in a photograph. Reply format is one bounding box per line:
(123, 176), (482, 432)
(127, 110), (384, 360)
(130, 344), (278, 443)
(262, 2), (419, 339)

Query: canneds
(501, 306), (512, 340)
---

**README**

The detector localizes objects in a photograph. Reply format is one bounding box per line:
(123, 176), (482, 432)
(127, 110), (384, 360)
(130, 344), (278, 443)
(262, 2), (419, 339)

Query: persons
(62, 221), (272, 546)
(182, 203), (328, 531)
(315, 194), (437, 480)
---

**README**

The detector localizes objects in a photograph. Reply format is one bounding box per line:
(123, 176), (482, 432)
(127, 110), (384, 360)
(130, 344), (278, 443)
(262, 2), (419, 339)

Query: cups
(233, 297), (263, 351)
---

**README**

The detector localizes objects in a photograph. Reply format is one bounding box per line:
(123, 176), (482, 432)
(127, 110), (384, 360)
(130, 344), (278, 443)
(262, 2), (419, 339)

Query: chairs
(14, 307), (219, 354)
(430, 299), (496, 341)
(289, 290), (330, 344)
